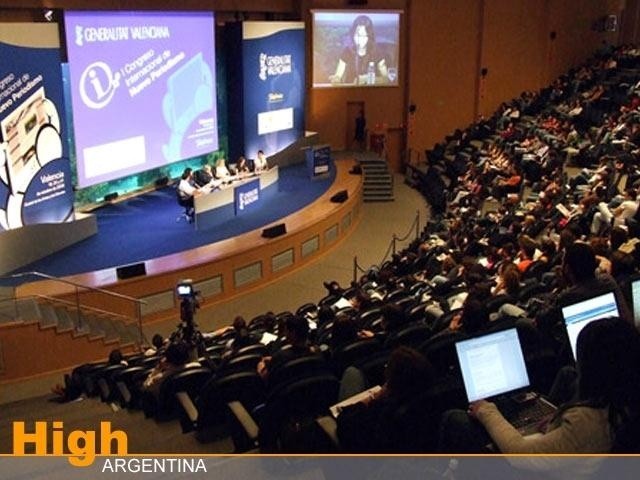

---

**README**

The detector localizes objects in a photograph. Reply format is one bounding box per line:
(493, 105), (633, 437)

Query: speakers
(481, 68), (487, 75)
(261, 222), (288, 238)
(410, 104), (416, 112)
(116, 263), (146, 277)
(550, 32), (556, 40)
(330, 189), (349, 203)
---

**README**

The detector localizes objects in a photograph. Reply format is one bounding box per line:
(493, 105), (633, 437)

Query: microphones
(351, 28), (362, 84)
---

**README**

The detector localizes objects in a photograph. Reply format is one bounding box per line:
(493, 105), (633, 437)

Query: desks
(191, 165), (280, 232)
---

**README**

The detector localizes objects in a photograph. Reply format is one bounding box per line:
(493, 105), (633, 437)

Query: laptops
(558, 288), (622, 365)
(455, 325), (559, 438)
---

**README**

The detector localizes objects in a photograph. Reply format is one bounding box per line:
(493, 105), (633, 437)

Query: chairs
(90, 279), (550, 419)
(176, 188), (193, 224)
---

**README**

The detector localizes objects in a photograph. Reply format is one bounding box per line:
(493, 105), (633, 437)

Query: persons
(327, 15), (396, 84)
